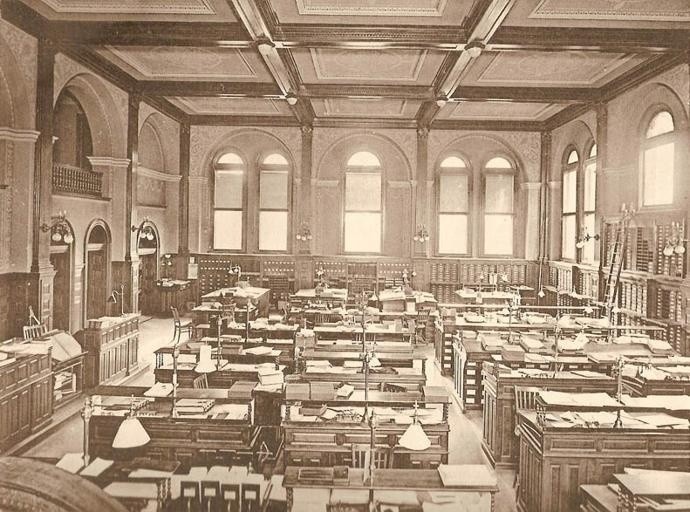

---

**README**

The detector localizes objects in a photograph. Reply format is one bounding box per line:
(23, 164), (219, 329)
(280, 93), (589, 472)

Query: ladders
(599, 221), (629, 321)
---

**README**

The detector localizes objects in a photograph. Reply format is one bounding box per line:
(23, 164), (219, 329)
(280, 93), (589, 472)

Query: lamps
(254, 33), (275, 56)
(662, 221), (689, 256)
(296, 220), (312, 242)
(413, 223), (430, 243)
(132, 215), (154, 241)
(284, 90), (297, 106)
(575, 226), (599, 249)
(42, 210), (73, 244)
(434, 97), (449, 108)
(464, 42), (485, 59)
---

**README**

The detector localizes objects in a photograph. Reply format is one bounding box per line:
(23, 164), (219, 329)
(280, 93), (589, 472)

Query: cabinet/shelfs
(0, 335), (54, 457)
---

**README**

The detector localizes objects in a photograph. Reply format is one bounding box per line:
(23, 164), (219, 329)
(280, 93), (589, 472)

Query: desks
(80, 285), (690, 512)
